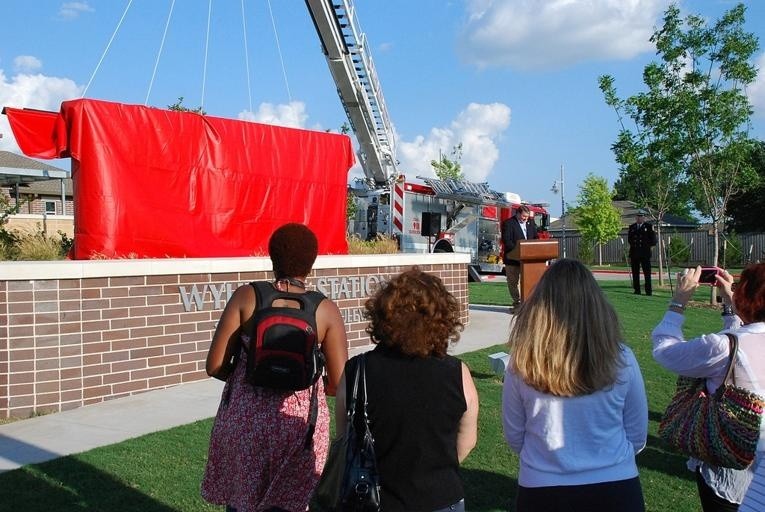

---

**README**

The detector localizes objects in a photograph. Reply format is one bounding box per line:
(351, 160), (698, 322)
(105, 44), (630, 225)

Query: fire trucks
(306, 0), (551, 279)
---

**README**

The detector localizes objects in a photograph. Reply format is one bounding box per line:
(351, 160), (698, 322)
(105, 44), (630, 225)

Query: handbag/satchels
(656, 333), (765, 473)
(306, 352), (384, 512)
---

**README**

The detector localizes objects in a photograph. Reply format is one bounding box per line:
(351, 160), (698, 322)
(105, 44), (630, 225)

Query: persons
(334, 266), (480, 512)
(199, 221), (348, 512)
(502, 258), (649, 511)
(500, 206), (539, 311)
(626, 209), (658, 297)
(651, 260), (765, 511)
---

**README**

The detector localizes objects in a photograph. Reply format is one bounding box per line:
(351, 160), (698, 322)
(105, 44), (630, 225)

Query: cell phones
(696, 268), (717, 285)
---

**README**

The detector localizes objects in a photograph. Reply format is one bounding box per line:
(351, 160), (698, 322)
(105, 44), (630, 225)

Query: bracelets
(722, 302), (734, 315)
(669, 301), (686, 311)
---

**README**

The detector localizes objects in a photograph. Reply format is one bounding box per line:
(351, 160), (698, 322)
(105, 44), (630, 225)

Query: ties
(639, 224), (640, 230)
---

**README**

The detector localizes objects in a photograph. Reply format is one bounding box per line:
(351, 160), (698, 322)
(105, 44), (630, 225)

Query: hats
(634, 209), (647, 216)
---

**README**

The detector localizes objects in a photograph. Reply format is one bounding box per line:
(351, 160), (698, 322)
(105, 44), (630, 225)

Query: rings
(681, 271), (687, 275)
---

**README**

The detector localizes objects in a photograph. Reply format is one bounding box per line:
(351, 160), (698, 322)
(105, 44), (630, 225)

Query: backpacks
(244, 280), (327, 391)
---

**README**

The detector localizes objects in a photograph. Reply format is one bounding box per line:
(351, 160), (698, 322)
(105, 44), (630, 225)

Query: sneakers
(634, 289), (652, 296)
